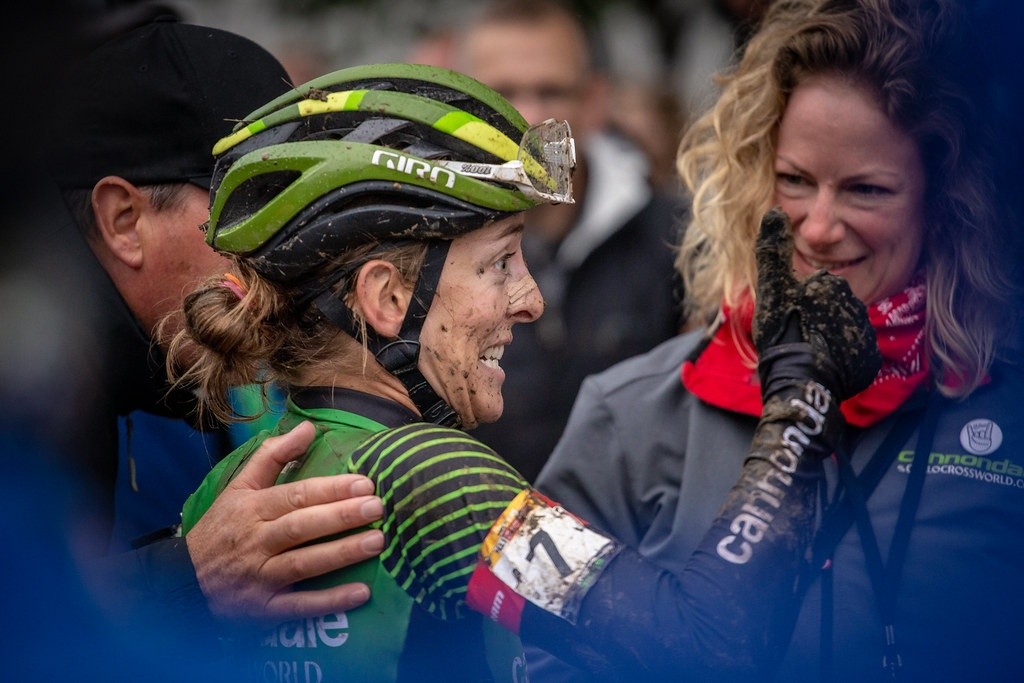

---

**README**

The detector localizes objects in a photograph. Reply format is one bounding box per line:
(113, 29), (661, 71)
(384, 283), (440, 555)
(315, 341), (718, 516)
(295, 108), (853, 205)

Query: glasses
(515, 118), (577, 204)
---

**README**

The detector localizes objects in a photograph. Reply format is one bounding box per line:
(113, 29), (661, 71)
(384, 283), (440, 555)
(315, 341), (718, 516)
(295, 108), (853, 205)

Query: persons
(525, 0), (1024, 683)
(0, 25), (384, 683)
(462, 1), (693, 485)
(156, 63), (880, 683)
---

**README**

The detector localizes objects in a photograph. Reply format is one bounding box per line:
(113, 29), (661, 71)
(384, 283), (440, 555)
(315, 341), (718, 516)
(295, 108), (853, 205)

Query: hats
(40, 16), (295, 191)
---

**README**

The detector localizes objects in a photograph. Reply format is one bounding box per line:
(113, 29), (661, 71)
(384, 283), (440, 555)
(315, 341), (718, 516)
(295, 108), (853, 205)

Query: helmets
(205, 69), (554, 284)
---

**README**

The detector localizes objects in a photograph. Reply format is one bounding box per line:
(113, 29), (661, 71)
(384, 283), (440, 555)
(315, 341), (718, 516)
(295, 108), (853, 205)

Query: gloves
(752, 208), (883, 406)
